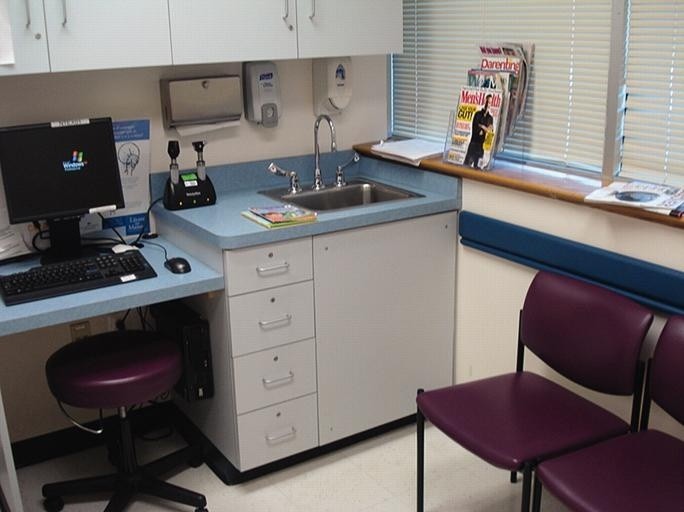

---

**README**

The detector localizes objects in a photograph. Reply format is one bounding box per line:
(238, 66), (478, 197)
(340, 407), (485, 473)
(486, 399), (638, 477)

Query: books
(371, 138), (445, 167)
(584, 181), (684, 218)
(249, 203), (317, 223)
(80, 118), (151, 240)
(241, 211), (319, 229)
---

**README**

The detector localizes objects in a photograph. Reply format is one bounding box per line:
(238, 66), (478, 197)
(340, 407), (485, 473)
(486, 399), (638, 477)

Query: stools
(37, 324), (213, 512)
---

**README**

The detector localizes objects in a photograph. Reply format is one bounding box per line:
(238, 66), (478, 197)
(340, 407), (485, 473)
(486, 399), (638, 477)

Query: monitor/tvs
(1, 116), (126, 266)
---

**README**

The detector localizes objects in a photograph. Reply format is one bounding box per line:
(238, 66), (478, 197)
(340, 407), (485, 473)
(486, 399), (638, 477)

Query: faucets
(313, 114), (338, 193)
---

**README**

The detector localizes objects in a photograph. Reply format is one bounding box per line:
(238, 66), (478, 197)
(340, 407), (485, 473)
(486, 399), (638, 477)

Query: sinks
(258, 175), (426, 217)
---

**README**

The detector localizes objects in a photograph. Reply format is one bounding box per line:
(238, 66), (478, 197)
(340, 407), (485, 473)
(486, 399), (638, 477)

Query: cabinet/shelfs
(169, 0), (404, 67)
(223, 213), (459, 472)
(0, 0), (172, 76)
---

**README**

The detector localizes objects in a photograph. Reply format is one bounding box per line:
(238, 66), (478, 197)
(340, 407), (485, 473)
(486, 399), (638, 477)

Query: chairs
(412, 267), (654, 511)
(529, 316), (683, 512)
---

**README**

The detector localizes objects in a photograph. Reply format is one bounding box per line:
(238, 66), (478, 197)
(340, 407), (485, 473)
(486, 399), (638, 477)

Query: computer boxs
(156, 305), (215, 404)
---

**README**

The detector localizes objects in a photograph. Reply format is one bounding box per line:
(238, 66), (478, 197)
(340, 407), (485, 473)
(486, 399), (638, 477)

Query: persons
(447, 40), (535, 172)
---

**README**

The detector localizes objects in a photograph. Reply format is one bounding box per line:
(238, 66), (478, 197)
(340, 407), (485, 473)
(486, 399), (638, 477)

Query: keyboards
(1, 250), (158, 306)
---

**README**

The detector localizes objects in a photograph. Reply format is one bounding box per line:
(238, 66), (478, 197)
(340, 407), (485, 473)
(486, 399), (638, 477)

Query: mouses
(163, 257), (192, 275)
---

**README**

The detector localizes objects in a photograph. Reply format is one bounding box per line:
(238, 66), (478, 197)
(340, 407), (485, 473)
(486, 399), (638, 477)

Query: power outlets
(108, 313), (126, 332)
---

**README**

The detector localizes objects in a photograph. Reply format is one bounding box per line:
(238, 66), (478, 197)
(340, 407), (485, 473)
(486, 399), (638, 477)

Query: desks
(1, 232), (227, 512)
